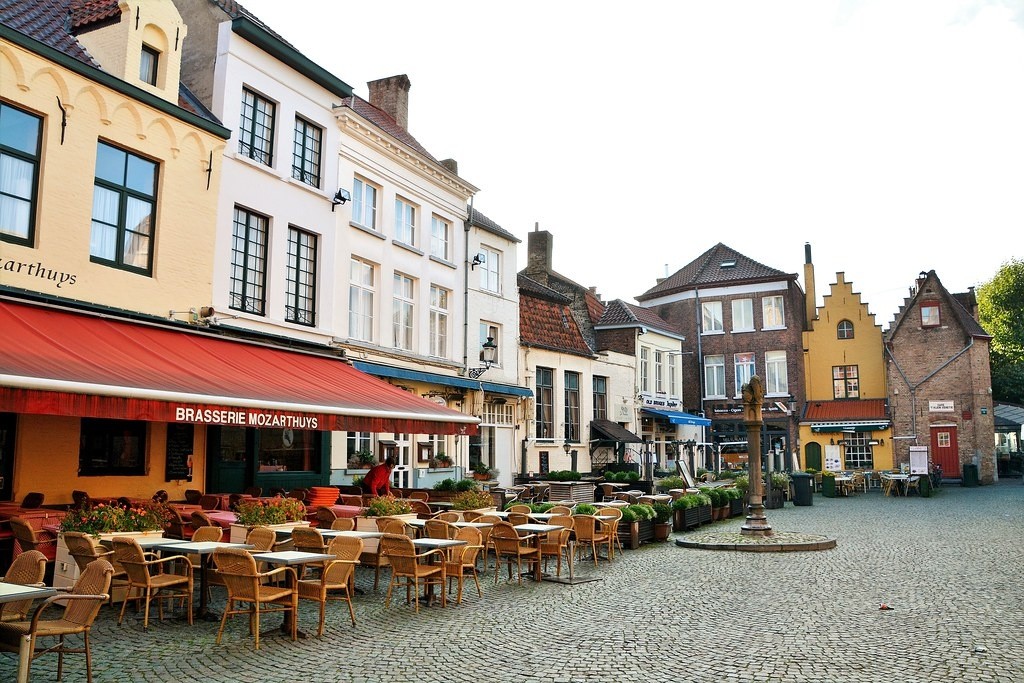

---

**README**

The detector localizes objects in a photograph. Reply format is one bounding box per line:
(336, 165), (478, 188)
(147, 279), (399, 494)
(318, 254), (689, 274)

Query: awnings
(0, 297), (482, 435)
(351, 360), (533, 397)
(589, 420), (644, 453)
(642, 408), (712, 426)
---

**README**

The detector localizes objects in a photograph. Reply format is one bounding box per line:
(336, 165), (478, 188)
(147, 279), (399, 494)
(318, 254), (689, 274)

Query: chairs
(814, 469), (921, 497)
(475, 480), (672, 504)
(0, 489), (622, 683)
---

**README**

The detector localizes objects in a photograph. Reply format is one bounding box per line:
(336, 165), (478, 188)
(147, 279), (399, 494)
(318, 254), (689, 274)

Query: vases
(354, 513), (418, 567)
(53, 529), (164, 607)
(228, 520), (311, 583)
(823, 476), (836, 497)
(458, 505), (496, 521)
(770, 484), (785, 508)
(809, 475), (816, 493)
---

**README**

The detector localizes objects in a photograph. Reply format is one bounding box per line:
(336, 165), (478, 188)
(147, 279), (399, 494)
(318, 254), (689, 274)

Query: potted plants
(503, 501), (673, 550)
(656, 476), (683, 491)
(472, 462), (500, 480)
(673, 488), (745, 531)
(599, 471), (653, 494)
(429, 451), (453, 469)
(351, 449), (379, 470)
(547, 470), (582, 480)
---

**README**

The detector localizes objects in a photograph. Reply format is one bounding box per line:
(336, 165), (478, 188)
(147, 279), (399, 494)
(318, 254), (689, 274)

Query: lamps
(332, 188), (351, 212)
(638, 327), (647, 337)
(472, 253), (485, 270)
(563, 442), (573, 455)
(894, 388), (899, 395)
(830, 437), (834, 445)
(837, 440), (848, 446)
(881, 439), (884, 446)
(469, 337), (498, 379)
(867, 439), (879, 446)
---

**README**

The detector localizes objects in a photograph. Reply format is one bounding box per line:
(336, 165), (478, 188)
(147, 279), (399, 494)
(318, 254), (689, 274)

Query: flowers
(821, 469), (835, 476)
(449, 490), (494, 510)
(233, 494), (309, 527)
(806, 468), (818, 474)
(771, 473), (786, 484)
(55, 492), (177, 541)
(359, 491), (414, 520)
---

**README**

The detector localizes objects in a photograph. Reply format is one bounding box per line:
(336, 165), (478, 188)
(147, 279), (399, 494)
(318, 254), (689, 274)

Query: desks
(599, 482), (630, 491)
(513, 523), (565, 577)
(100, 537), (189, 608)
(482, 511), (509, 519)
(0, 501), (68, 566)
(173, 503), (202, 510)
(92, 496), (152, 509)
(396, 497), (422, 503)
(340, 494), (363, 506)
(158, 541), (256, 623)
(405, 519), (427, 563)
(448, 522), (494, 573)
(323, 530), (390, 595)
(428, 501), (454, 510)
(834, 476), (851, 496)
(526, 512), (564, 523)
(177, 508), (241, 533)
(636, 495), (673, 501)
(201, 493), (253, 511)
(410, 538), (468, 608)
(523, 483), (549, 488)
(582, 515), (618, 561)
(275, 528), (337, 580)
(331, 504), (369, 518)
(0, 582), (57, 619)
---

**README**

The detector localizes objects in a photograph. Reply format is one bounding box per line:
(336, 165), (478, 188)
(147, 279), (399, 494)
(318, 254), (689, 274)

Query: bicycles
(928, 461), (943, 486)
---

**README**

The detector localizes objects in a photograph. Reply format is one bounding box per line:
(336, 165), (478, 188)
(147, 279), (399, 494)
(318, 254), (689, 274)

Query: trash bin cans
(963, 463), (979, 487)
(1001, 460), (1012, 474)
(791, 472), (814, 506)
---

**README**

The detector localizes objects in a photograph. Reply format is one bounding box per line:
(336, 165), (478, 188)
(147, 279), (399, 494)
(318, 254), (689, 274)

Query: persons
(360, 457), (396, 498)
